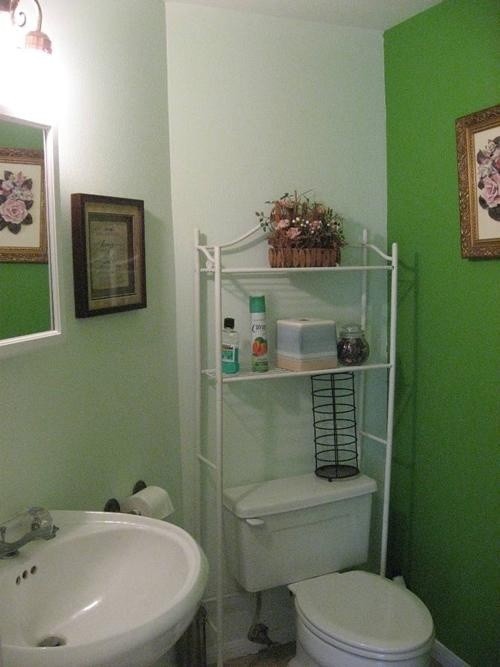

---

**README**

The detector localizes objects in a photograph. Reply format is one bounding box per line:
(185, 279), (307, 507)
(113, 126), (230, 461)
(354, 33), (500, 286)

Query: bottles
(337, 324), (370, 366)
(249, 296), (268, 373)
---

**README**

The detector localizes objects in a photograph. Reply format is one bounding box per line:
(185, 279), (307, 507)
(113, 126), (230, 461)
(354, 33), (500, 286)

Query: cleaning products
(221, 318), (240, 374)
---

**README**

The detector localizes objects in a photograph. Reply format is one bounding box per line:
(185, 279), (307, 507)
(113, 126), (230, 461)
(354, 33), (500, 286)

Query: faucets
(0, 507), (56, 553)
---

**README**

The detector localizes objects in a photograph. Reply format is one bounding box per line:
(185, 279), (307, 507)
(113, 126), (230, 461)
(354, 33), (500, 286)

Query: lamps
(0, 0), (51, 57)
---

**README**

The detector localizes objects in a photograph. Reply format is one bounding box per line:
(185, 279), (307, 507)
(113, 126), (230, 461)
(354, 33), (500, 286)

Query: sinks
(0, 508), (208, 667)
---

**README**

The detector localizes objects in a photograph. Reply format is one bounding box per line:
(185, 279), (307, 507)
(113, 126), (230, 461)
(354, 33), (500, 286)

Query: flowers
(258, 192), (352, 248)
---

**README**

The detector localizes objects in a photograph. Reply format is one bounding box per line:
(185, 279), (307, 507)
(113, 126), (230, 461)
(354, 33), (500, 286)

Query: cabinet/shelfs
(189, 212), (402, 667)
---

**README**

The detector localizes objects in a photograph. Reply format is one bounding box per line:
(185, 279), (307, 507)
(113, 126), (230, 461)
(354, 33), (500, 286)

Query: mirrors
(2, 111), (61, 347)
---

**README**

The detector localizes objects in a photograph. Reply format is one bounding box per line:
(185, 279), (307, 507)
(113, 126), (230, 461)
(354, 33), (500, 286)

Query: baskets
(267, 248), (341, 268)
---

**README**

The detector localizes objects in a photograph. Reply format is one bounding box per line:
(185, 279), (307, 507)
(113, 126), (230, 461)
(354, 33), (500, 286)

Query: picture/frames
(454, 104), (500, 259)
(70, 192), (147, 316)
(0, 145), (49, 265)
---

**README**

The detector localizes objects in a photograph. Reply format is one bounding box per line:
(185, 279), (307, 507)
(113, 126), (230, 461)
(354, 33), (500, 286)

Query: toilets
(221, 473), (436, 665)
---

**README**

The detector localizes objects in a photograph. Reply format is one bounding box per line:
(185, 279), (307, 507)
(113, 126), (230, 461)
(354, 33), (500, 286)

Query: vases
(268, 239), (341, 266)
